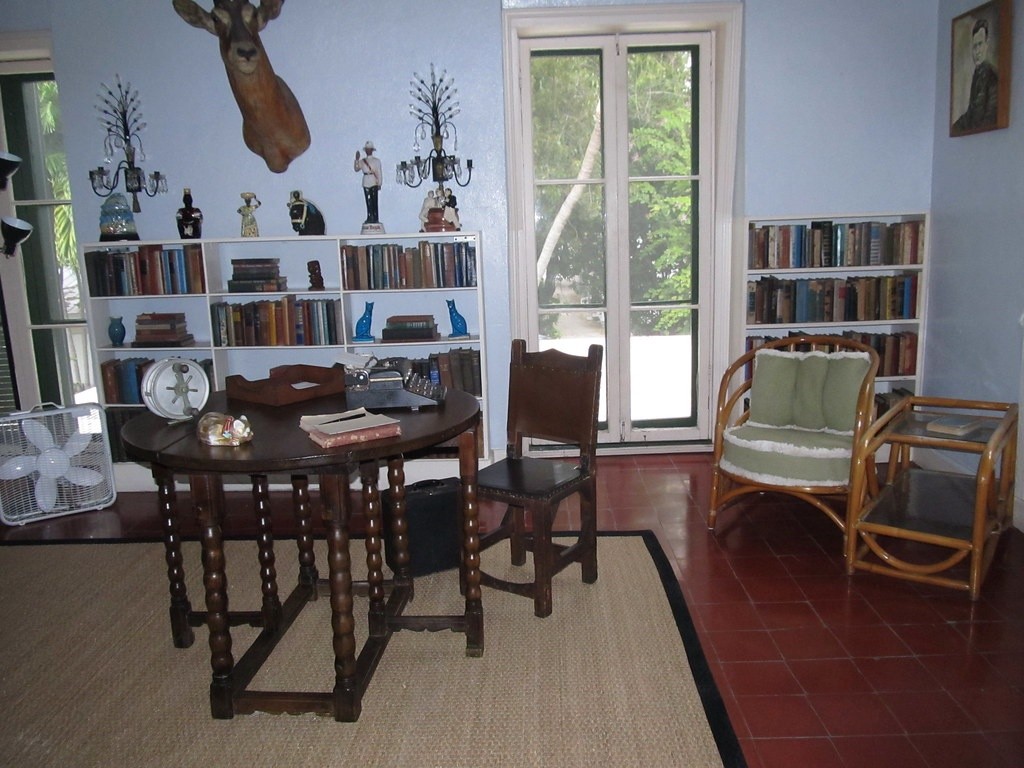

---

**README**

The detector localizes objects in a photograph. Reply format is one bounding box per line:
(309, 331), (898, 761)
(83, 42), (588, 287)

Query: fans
(0, 402), (117, 524)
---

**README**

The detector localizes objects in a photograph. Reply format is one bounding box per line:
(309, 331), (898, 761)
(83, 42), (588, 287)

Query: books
(749, 220), (925, 437)
(132, 312), (195, 348)
(411, 347), (482, 397)
(211, 295), (344, 347)
(228, 259), (287, 291)
(300, 406), (401, 448)
(85, 245), (206, 297)
(381, 315), (441, 342)
(101, 357), (216, 404)
(341, 240), (477, 291)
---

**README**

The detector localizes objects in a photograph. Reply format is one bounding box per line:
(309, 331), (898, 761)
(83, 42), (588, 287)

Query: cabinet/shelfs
(80, 230), (490, 492)
(743, 209), (930, 409)
(846, 395), (1019, 601)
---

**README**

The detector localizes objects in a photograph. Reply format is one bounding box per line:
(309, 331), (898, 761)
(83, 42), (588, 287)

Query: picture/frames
(949, 0), (1010, 137)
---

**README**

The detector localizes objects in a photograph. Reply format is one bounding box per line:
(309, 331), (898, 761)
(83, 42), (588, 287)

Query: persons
(419, 190), (435, 232)
(237, 195), (261, 237)
(443, 188), (461, 231)
(354, 141), (383, 223)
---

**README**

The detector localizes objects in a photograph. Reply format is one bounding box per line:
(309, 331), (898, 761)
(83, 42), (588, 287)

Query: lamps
(88, 75), (169, 212)
(396, 63), (474, 193)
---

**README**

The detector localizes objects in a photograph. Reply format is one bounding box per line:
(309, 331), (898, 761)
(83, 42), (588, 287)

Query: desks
(119, 388), (485, 722)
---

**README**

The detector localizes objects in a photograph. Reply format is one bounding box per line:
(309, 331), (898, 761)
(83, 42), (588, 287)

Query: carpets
(0, 528), (746, 768)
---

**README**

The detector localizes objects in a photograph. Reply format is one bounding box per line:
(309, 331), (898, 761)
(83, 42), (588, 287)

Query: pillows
(748, 349), (871, 435)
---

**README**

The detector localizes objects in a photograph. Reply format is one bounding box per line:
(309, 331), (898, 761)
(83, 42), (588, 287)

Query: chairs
(460, 339), (602, 616)
(707, 334), (880, 556)
